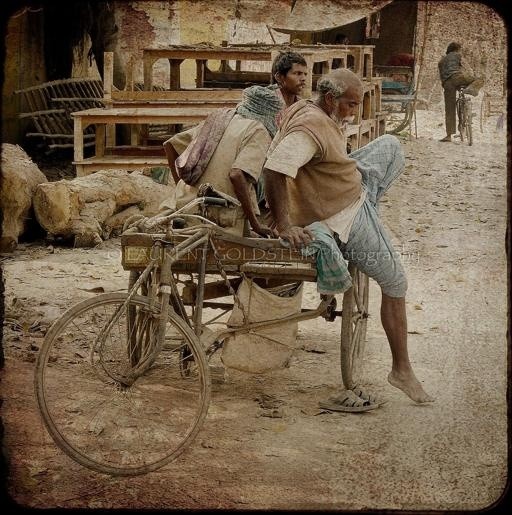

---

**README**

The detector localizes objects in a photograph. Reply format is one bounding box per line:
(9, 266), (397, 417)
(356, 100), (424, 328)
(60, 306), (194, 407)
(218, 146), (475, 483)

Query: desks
(68, 43), (393, 178)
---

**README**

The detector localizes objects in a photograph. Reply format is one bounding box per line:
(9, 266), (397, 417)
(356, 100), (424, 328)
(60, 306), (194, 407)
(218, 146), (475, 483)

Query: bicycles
(453, 85), (476, 146)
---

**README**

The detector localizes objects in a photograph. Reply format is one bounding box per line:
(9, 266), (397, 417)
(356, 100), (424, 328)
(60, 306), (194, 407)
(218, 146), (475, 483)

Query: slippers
(318, 389), (379, 413)
(329, 388), (389, 406)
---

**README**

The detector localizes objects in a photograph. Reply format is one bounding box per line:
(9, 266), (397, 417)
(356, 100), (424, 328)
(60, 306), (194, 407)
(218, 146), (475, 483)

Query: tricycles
(34, 182), (370, 479)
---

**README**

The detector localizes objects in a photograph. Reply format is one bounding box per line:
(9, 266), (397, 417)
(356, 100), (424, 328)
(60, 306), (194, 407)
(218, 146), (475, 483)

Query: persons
(438, 42), (484, 142)
(264, 68), (436, 402)
(270, 52), (307, 126)
(163, 85), (286, 238)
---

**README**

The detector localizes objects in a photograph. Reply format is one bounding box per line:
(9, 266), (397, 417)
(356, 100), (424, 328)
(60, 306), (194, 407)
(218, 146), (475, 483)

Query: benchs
(11, 72), (172, 154)
